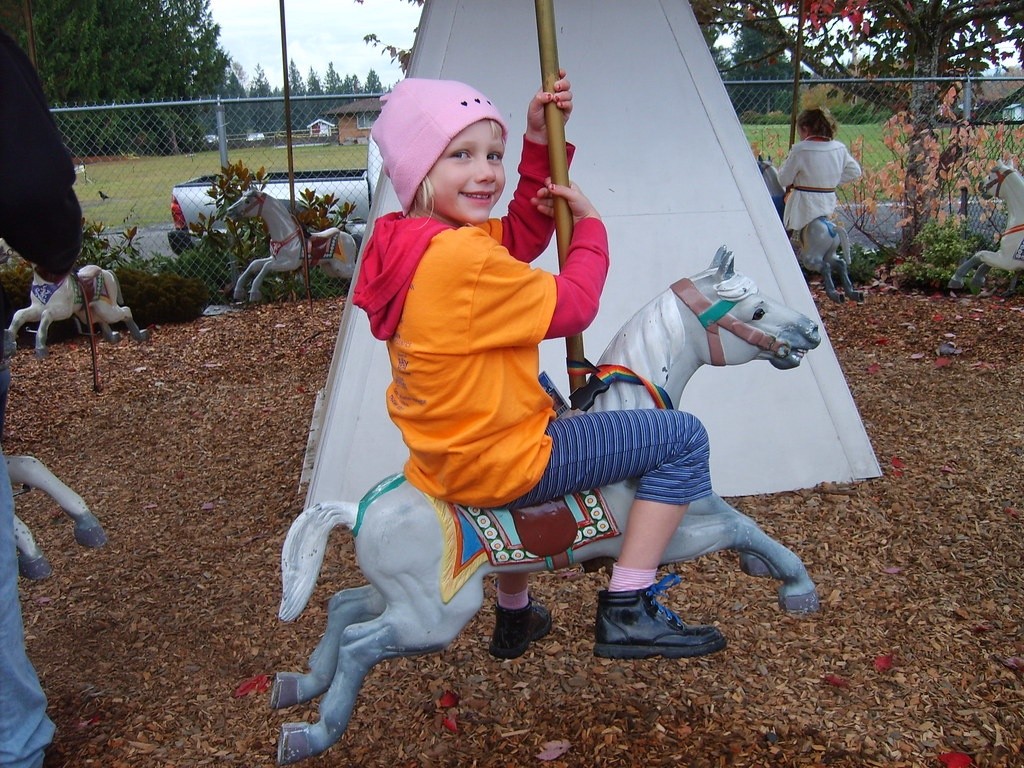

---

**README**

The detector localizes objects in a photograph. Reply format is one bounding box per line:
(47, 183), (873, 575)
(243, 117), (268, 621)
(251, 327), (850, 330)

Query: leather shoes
(593, 574), (726, 658)
(488, 597), (552, 660)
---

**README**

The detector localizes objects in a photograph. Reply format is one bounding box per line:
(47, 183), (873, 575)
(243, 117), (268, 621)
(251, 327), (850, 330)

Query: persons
(771, 106), (862, 232)
(0, 27), (84, 768)
(353, 69), (727, 660)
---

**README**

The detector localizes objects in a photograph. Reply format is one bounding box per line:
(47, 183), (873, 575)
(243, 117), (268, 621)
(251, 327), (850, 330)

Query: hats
(371, 78), (507, 217)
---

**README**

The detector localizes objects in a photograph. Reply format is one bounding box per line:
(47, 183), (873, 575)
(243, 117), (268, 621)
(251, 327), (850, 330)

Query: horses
(225, 189), (363, 303)
(1, 456), (107, 580)
(8, 262), (148, 361)
(755, 154), (1023, 305)
(269, 245), (823, 765)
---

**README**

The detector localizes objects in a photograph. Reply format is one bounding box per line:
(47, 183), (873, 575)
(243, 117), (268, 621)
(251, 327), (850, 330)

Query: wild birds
(98, 191), (110, 201)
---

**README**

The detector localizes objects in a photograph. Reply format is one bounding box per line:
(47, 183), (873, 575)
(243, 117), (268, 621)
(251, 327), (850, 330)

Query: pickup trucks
(167, 136), (383, 257)
